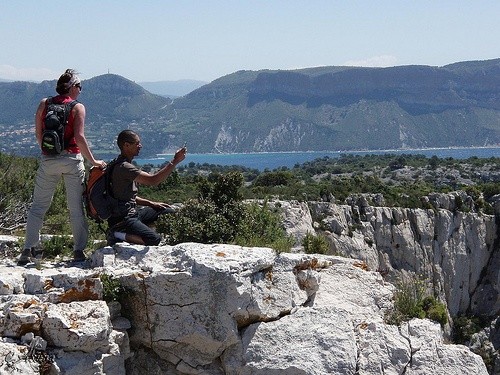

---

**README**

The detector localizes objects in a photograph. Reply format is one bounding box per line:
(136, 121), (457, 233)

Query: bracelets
(169, 160), (175, 168)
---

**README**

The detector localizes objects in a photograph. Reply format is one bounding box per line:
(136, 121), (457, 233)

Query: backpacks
(84, 158), (138, 232)
(41, 96), (81, 155)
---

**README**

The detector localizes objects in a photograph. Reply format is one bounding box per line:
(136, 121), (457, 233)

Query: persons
(18, 68), (107, 264)
(85, 129), (187, 245)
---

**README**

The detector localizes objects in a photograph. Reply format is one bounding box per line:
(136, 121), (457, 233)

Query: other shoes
(74, 250), (87, 262)
(17, 248), (31, 266)
(106, 230), (123, 247)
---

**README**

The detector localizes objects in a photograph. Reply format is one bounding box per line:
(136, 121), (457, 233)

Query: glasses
(127, 141), (140, 146)
(75, 84), (82, 90)
